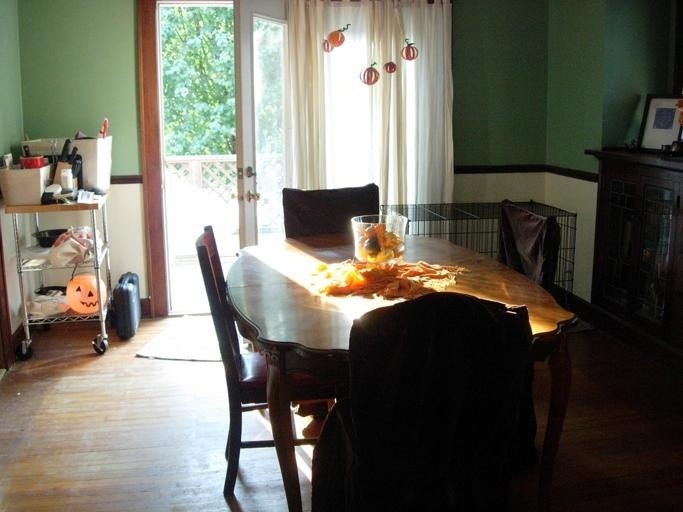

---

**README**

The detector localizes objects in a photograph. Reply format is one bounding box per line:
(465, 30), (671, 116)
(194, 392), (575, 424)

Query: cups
(18, 154), (45, 169)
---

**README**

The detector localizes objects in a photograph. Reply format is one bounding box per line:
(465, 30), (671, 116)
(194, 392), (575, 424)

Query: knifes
(58, 139), (84, 178)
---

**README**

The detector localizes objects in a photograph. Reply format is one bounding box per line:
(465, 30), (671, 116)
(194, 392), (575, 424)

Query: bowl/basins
(32, 229), (68, 248)
(351, 214), (408, 263)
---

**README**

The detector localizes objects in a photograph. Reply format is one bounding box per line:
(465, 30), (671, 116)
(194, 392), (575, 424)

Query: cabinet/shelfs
(4, 203), (114, 358)
(585, 149), (681, 362)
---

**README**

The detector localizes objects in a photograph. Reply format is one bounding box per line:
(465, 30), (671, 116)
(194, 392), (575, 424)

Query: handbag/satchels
(21, 136), (113, 195)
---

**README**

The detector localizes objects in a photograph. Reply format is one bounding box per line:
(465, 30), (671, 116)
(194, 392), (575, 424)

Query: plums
(364, 239), (377, 253)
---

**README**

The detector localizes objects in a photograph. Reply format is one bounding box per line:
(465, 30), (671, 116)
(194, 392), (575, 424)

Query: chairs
(198, 223), (341, 511)
(498, 197), (560, 291)
(283, 182), (381, 237)
(325, 290), (541, 511)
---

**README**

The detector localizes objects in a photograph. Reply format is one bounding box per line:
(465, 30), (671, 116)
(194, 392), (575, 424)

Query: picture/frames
(637, 91), (681, 151)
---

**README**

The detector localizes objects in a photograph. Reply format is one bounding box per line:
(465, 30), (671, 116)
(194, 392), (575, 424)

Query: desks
(221, 231), (579, 512)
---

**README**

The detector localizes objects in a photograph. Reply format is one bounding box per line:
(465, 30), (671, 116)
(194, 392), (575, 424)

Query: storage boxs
(2, 131), (113, 205)
